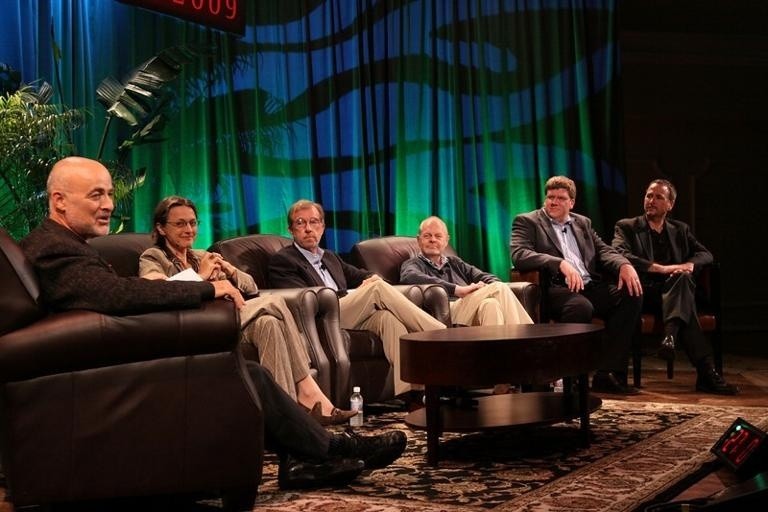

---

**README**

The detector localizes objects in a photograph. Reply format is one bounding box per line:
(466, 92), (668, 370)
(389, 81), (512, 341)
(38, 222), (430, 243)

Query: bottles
(349, 387), (363, 426)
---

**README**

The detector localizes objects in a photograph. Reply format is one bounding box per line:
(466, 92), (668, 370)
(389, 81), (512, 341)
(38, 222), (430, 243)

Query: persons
(266, 198), (448, 413)
(395, 214), (537, 327)
(18, 155), (407, 488)
(609, 177), (742, 396)
(138, 195), (361, 426)
(510, 176), (647, 395)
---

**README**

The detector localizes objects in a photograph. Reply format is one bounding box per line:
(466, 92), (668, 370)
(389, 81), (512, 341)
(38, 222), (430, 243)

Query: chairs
(620, 263), (727, 391)
(87, 234), (331, 401)
(1, 234), (262, 509)
(344, 236), (543, 327)
(509, 268), (612, 328)
(215, 234), (425, 402)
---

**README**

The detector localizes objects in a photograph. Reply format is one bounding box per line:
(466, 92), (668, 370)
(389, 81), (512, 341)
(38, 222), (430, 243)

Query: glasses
(291, 218), (321, 227)
(165, 220), (198, 228)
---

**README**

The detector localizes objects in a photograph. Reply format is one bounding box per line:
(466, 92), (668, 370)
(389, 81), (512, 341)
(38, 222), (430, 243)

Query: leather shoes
(342, 428), (405, 469)
(659, 333), (676, 359)
(279, 455), (364, 488)
(696, 368), (740, 394)
(592, 371), (641, 395)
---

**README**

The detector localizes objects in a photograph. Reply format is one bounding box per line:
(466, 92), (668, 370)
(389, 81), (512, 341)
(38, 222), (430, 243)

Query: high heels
(311, 403), (323, 421)
(298, 400), (359, 427)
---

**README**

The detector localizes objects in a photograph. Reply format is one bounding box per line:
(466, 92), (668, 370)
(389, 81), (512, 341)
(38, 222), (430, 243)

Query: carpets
(168, 393), (768, 512)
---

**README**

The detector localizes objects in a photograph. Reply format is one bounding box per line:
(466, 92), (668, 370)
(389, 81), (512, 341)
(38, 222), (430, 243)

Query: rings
(635, 279), (640, 282)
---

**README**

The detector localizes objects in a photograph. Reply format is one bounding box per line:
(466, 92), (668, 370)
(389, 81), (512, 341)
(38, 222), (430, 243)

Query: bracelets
(230, 269), (237, 279)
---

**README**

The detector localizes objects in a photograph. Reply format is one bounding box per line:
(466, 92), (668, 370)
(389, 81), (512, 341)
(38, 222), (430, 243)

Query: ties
(560, 223), (584, 279)
